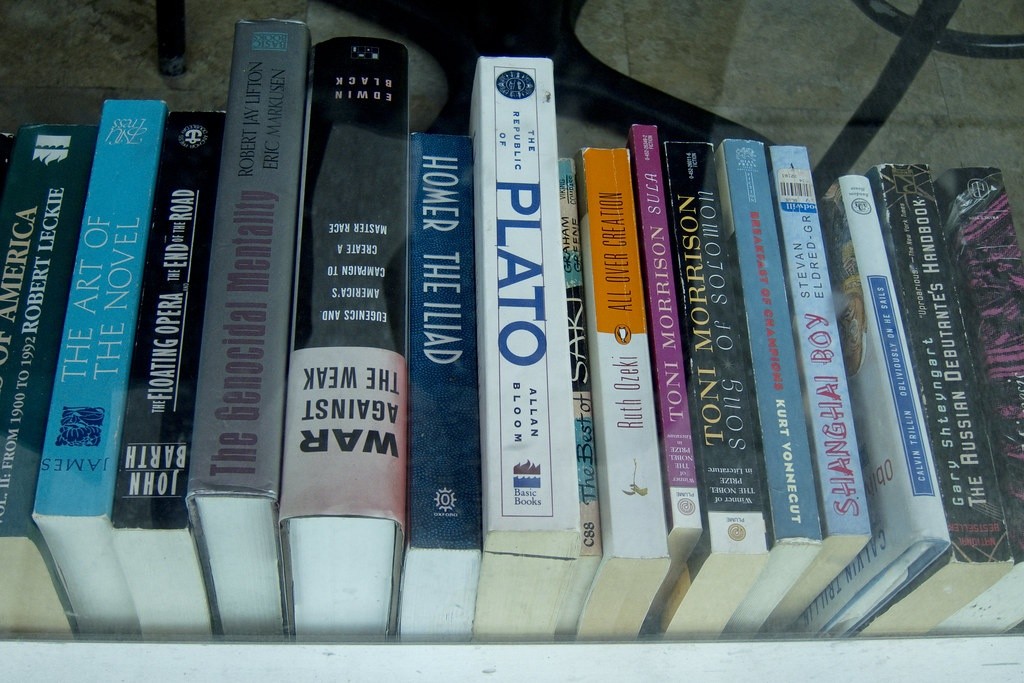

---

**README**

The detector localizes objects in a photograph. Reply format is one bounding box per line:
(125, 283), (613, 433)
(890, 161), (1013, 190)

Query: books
(1, 19), (1024, 639)
